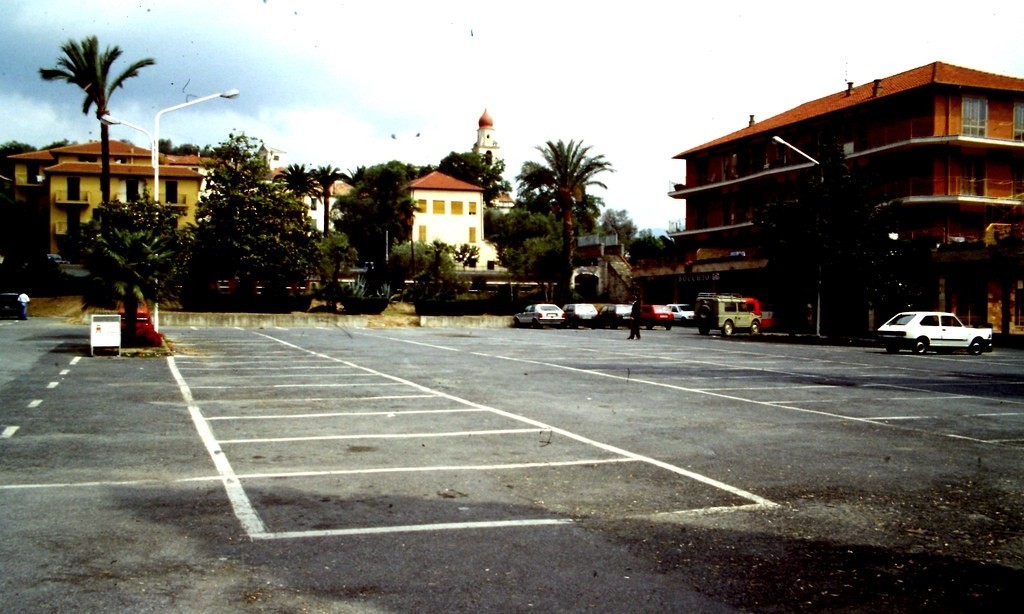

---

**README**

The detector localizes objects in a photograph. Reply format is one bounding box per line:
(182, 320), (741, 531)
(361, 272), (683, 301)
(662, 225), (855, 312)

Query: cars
(665, 303), (696, 325)
(877, 311), (993, 356)
(0, 291), (29, 321)
(561, 302), (600, 328)
(513, 304), (567, 328)
(598, 304), (636, 329)
(114, 300), (152, 323)
(639, 304), (675, 330)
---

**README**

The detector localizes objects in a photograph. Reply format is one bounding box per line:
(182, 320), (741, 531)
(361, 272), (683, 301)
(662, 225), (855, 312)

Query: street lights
(100, 87), (243, 335)
(770, 135), (823, 338)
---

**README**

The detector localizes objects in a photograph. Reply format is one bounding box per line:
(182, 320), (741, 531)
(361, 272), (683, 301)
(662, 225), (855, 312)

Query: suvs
(696, 292), (762, 335)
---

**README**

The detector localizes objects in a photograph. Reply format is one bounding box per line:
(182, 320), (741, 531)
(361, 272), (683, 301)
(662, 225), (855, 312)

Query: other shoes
(627, 336), (642, 341)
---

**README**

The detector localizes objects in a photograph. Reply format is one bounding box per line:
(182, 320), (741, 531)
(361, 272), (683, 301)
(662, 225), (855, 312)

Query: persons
(17, 290), (31, 321)
(627, 293), (642, 340)
